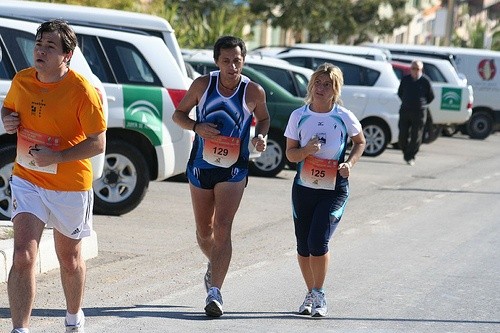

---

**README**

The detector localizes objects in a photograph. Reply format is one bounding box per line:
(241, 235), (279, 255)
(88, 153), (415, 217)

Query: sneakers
(311, 291), (328, 317)
(204, 289), (224, 317)
(204, 261), (213, 294)
(299, 293), (313, 315)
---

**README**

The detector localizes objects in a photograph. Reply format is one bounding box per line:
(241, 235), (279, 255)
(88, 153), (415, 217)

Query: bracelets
(193, 122), (199, 131)
(347, 160), (352, 168)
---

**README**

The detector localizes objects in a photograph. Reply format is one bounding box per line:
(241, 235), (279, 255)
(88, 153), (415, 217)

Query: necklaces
(219, 79), (239, 90)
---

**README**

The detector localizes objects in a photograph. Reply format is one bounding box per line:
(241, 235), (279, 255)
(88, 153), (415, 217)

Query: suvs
(0, 0), (197, 221)
(386, 47), (474, 141)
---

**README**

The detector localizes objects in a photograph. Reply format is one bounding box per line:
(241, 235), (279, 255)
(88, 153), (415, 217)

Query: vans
(360, 41), (500, 140)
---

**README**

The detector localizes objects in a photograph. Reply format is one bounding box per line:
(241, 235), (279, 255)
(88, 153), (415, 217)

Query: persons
(284, 62), (367, 318)
(172, 36), (271, 317)
(1, 18), (108, 333)
(397, 60), (435, 165)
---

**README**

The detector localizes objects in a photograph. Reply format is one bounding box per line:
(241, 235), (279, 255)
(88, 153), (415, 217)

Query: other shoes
(408, 159), (415, 165)
(64, 311), (84, 333)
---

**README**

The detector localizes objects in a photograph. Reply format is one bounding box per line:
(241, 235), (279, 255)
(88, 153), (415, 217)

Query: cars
(182, 44), (403, 178)
